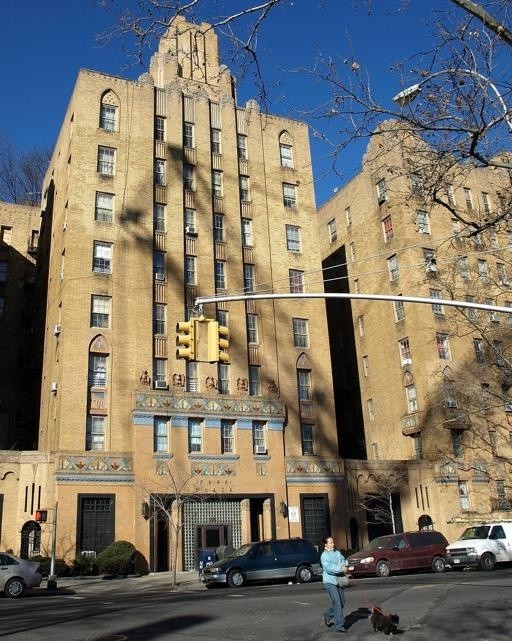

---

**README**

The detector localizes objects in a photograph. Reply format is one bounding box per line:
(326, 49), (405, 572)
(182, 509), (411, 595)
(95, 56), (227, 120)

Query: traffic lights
(173, 315), (197, 362)
(35, 509), (48, 523)
(207, 318), (232, 367)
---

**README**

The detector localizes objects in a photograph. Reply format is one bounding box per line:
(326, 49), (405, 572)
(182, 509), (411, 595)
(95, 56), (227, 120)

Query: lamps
(279, 499), (286, 513)
(140, 500), (148, 516)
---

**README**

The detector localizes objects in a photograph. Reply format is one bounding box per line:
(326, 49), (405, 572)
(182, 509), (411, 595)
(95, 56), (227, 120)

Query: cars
(0, 550), (43, 600)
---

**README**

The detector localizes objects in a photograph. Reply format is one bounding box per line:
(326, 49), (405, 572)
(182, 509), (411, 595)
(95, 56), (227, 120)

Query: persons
(320, 537), (351, 634)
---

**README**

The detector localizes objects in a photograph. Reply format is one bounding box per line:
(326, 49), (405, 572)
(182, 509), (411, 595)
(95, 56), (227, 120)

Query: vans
(344, 530), (452, 575)
(200, 536), (321, 585)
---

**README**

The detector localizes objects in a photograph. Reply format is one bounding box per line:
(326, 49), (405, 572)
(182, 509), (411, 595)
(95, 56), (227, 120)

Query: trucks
(446, 519), (511, 571)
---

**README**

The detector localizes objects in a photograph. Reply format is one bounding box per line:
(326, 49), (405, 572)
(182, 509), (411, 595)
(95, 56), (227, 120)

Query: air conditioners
(154, 378), (168, 390)
(186, 225), (197, 234)
(155, 271), (165, 281)
(51, 323), (61, 336)
(49, 381), (57, 392)
(490, 314), (501, 322)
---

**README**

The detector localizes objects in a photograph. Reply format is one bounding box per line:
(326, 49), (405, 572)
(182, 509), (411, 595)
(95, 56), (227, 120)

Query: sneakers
(322, 610), (333, 628)
(336, 628), (347, 633)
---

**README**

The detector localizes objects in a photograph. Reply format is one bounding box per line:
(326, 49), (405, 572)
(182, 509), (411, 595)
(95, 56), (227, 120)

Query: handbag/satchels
(337, 563), (351, 588)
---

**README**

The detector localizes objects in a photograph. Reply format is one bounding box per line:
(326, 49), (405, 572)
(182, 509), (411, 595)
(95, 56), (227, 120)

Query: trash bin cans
(197, 546), (234, 579)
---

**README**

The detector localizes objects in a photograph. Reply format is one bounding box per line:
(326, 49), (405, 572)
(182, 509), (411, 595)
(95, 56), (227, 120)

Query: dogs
(370, 606), (399, 637)
